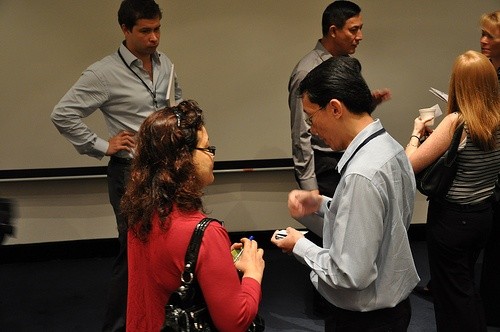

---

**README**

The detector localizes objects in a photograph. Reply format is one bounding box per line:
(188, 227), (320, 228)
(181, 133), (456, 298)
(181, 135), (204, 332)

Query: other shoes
(303, 286), (328, 321)
(412, 286), (434, 303)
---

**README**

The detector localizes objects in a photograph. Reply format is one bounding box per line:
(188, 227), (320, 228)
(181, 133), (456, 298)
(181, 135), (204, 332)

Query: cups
(418, 108), (436, 126)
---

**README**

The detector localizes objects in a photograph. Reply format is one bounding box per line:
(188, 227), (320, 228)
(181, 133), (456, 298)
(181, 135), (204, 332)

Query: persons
(286, 0), (392, 322)
(478, 6), (500, 69)
(269, 56), (422, 332)
(51, 0), (182, 331)
(404, 49), (500, 332)
(124, 100), (265, 332)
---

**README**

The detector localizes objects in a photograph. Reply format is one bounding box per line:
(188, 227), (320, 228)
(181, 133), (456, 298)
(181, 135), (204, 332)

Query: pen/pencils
(234, 235), (254, 262)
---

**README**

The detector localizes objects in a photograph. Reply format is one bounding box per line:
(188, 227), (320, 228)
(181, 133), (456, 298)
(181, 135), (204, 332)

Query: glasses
(195, 145), (216, 157)
(304, 98), (342, 126)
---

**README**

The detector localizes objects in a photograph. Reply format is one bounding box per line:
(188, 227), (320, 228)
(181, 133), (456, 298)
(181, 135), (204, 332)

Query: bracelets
(409, 132), (423, 146)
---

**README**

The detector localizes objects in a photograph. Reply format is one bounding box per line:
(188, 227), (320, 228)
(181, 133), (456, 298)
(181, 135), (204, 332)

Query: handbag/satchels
(161, 218), (265, 332)
(413, 111), (465, 201)
